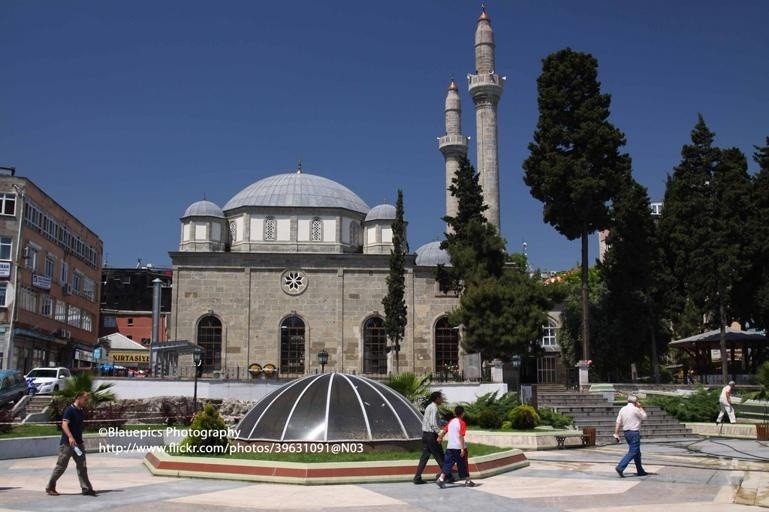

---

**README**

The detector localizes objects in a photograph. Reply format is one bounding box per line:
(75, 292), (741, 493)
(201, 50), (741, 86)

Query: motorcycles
(26, 376), (37, 396)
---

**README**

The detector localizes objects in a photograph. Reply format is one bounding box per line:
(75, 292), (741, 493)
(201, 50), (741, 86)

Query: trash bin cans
(588, 383), (617, 401)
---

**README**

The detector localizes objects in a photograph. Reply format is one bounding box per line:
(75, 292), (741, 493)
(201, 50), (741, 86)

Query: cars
(24, 366), (75, 396)
(99, 363), (153, 378)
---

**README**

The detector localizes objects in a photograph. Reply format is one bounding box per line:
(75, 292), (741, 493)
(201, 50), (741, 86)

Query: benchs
(553, 433), (592, 450)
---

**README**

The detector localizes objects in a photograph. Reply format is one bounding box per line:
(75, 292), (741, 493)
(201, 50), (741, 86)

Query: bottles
(73, 446), (83, 456)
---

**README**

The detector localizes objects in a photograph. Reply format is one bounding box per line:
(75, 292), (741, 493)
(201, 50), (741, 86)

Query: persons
(715, 380), (737, 423)
(612, 394), (648, 477)
(435, 405), (477, 488)
(682, 351), (709, 384)
(412, 391), (456, 484)
(44, 390), (97, 497)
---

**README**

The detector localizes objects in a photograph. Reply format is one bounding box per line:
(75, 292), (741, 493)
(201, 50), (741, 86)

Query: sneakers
(46, 487), (96, 496)
(616, 466), (648, 478)
(414, 476), (475, 489)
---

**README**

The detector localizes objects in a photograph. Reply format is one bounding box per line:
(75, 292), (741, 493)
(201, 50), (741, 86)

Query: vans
(0, 368), (29, 408)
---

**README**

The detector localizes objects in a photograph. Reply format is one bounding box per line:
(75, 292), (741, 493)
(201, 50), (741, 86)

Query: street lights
(318, 348), (329, 374)
(189, 346), (205, 425)
